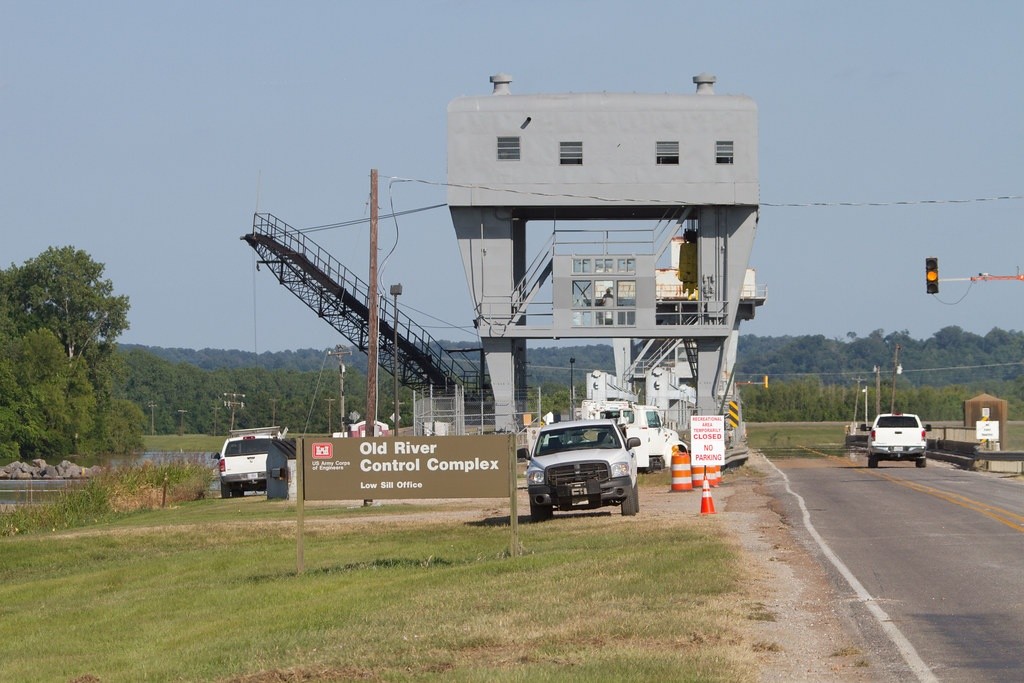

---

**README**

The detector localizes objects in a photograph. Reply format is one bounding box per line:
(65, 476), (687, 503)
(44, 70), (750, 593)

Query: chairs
(549, 437), (561, 450)
(597, 432), (615, 445)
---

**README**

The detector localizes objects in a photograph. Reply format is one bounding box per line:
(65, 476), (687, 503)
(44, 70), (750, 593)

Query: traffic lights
(925, 257), (939, 294)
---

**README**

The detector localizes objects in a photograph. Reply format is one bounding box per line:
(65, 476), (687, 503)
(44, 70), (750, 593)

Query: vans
(581, 397), (689, 474)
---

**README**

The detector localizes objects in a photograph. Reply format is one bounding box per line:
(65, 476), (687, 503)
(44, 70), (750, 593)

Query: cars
(865, 412), (932, 467)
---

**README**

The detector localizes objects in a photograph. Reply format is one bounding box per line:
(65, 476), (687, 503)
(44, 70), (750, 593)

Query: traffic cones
(700, 475), (716, 514)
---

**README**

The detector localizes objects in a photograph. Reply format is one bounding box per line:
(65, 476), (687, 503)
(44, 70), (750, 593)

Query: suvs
(515, 415), (642, 522)
(211, 432), (285, 499)
